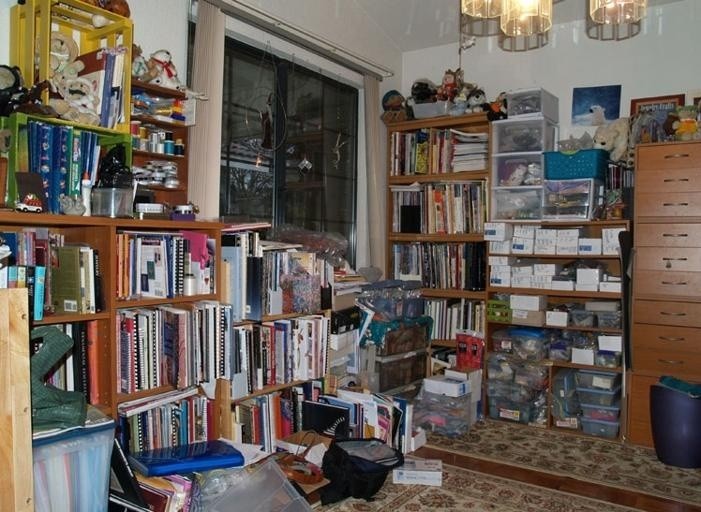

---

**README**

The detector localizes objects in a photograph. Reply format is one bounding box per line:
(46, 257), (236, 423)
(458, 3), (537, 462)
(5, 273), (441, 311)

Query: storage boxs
(574, 369), (622, 439)
(552, 367), (579, 417)
(32, 405), (116, 512)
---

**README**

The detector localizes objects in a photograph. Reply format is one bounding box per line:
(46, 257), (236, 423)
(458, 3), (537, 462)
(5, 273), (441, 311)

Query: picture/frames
(629, 93), (684, 144)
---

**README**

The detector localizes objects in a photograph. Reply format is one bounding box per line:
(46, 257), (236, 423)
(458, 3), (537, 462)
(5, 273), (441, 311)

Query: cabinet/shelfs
(484, 217), (631, 441)
(384, 110), (493, 416)
(629, 141), (700, 447)
(490, 117), (561, 220)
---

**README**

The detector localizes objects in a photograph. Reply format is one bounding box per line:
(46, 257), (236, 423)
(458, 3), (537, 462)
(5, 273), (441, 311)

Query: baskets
(542, 149), (609, 179)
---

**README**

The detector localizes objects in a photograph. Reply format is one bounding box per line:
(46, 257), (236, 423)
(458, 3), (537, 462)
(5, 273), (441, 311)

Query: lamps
(461, 0), (647, 38)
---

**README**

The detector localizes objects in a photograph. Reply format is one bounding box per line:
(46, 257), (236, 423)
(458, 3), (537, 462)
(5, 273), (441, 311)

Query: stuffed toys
(557, 104), (701, 163)
(0, 0), (182, 125)
(382, 69), (508, 122)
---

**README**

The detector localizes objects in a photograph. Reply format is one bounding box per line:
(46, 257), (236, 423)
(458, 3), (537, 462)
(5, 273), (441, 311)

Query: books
(390, 128), (486, 372)
(221, 223), (425, 456)
(1, 119), (244, 511)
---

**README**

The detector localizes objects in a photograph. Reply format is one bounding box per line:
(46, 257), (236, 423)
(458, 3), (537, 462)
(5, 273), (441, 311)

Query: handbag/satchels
(316, 437), (404, 507)
(258, 451), (324, 484)
